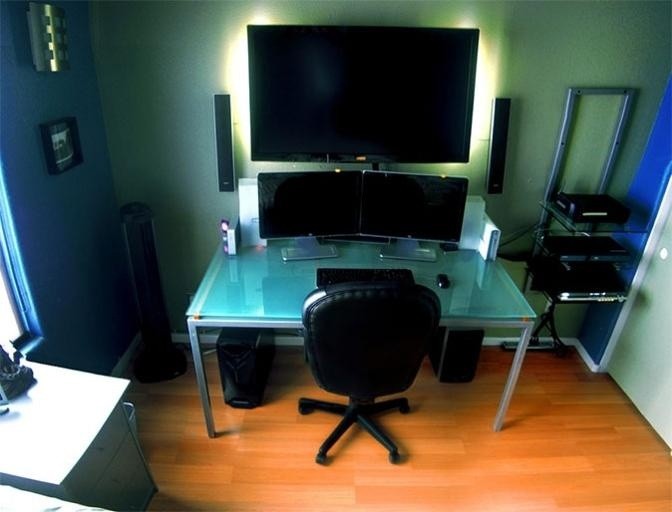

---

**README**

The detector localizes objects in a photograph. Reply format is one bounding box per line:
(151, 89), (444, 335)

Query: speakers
(118, 201), (188, 385)
(486, 96), (512, 196)
(210, 92), (237, 193)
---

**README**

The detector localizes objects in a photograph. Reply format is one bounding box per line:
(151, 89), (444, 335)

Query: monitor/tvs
(256, 171), (363, 262)
(245, 23), (480, 173)
(359, 171), (470, 264)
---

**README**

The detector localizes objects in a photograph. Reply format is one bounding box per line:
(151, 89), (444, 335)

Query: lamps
(27, 3), (72, 76)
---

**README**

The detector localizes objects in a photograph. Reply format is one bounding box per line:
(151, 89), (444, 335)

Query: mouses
(435, 272), (454, 291)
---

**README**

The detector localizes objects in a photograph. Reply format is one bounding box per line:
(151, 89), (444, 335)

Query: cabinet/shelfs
(535, 199), (649, 310)
(0, 361), (157, 509)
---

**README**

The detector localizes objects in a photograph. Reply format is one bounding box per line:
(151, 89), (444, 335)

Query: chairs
(297, 276), (440, 463)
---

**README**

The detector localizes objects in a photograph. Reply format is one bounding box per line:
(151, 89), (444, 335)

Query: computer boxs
(428, 324), (486, 384)
(215, 326), (278, 408)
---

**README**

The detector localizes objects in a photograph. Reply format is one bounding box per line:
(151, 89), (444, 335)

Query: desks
(185, 224), (536, 437)
